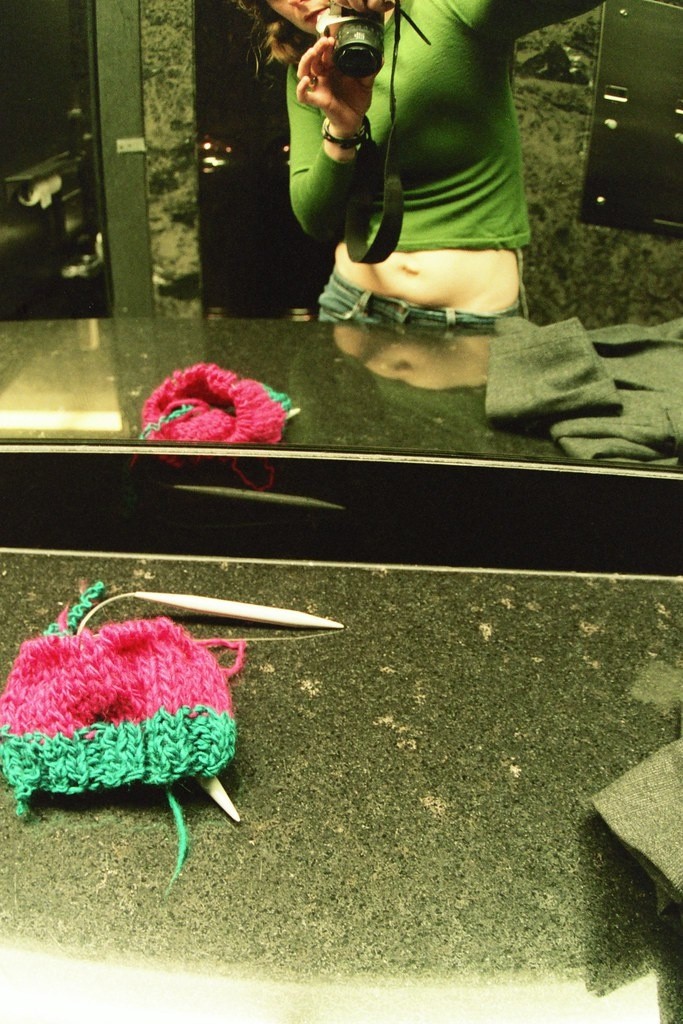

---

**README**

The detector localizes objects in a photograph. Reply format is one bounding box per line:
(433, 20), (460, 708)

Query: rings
(313, 77), (315, 81)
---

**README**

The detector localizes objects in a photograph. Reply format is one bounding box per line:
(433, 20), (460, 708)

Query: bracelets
(321, 116), (370, 150)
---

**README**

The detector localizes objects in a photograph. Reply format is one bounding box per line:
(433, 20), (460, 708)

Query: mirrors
(0, 0), (683, 577)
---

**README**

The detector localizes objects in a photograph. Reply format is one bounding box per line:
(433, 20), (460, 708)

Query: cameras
(315, 0), (386, 78)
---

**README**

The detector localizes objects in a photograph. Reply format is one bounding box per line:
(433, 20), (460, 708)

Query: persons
(235, 0), (605, 330)
